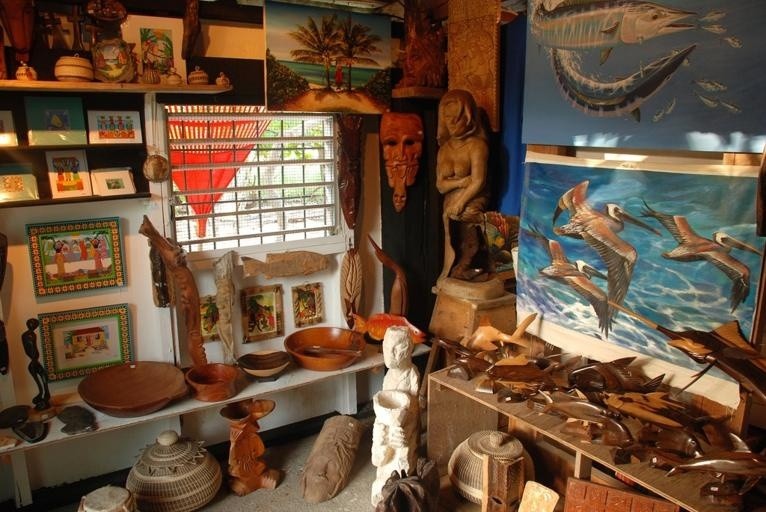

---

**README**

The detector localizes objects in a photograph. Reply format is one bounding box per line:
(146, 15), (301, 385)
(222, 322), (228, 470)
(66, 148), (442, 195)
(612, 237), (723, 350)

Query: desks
(415, 286), (517, 407)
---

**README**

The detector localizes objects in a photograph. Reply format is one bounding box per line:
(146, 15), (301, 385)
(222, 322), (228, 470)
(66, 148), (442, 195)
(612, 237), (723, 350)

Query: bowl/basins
(285, 327), (366, 370)
(77, 360), (184, 417)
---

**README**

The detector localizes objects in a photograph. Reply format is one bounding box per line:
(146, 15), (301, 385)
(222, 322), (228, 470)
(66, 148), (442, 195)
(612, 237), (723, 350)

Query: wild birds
(522, 180), (763, 340)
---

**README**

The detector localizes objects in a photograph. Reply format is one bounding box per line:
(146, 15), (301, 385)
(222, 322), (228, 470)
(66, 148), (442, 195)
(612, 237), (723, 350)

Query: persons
(435, 90), (493, 282)
(371, 325), (422, 509)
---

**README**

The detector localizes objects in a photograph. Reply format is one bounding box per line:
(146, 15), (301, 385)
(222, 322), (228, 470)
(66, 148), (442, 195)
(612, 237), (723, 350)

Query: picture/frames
(86, 109), (143, 144)
(199, 294), (221, 342)
(24, 93), (87, 145)
(90, 166), (136, 196)
(291, 282), (325, 329)
(0, 110), (18, 146)
(45, 148), (93, 199)
(25, 216), (128, 298)
(121, 14), (188, 87)
(0, 164), (40, 203)
(38, 303), (135, 384)
(240, 284), (285, 344)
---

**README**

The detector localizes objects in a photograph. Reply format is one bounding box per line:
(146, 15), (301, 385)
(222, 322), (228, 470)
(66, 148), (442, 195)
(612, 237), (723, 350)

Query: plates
(238, 351), (291, 381)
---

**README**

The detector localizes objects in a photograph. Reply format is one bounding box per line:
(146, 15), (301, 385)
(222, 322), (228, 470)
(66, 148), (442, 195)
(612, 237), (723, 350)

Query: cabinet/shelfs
(2, 321), (466, 506)
(425, 343), (766, 512)
